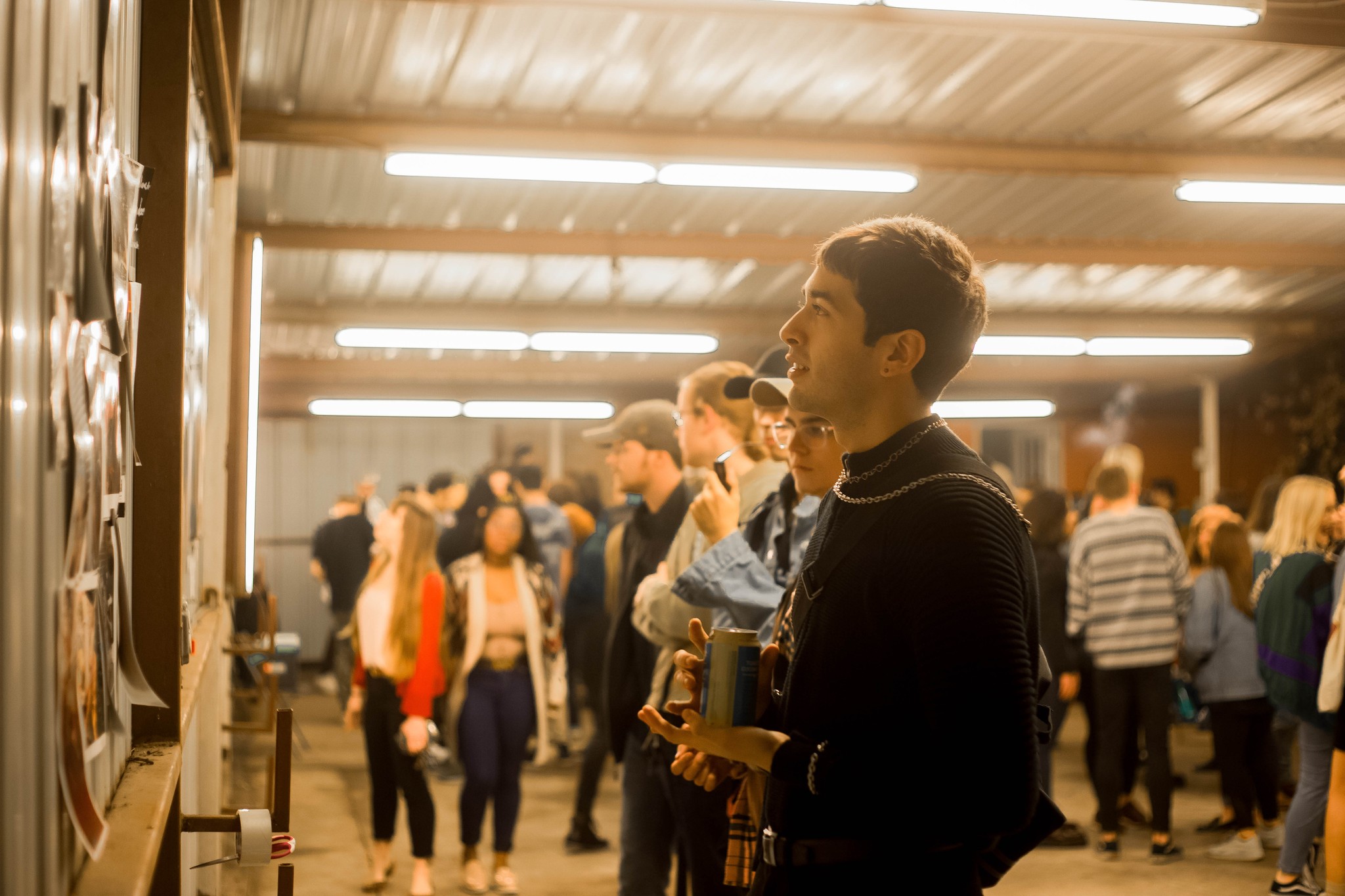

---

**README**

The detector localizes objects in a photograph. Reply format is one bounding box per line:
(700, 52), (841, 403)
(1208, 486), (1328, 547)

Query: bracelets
(808, 740), (828, 796)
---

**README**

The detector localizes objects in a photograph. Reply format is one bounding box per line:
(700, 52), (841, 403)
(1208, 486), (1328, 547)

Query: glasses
(673, 406), (710, 427)
(774, 422), (835, 450)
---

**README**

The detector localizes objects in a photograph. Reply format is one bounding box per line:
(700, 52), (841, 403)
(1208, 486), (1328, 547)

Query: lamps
(225, 235), (270, 601)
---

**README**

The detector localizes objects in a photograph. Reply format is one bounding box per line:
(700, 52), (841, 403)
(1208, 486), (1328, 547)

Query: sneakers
(1259, 824), (1285, 849)
(1205, 833), (1264, 862)
(1269, 876), (1323, 896)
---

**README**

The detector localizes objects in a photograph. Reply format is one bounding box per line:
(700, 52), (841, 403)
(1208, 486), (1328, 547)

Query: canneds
(698, 626), (762, 728)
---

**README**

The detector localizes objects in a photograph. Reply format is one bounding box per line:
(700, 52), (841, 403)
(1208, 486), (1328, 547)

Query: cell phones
(714, 450), (733, 494)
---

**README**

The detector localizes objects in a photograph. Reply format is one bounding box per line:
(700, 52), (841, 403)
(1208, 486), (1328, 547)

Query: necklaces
(833, 417), (1033, 535)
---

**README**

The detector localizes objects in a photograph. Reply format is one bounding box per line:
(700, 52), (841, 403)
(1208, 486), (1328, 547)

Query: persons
(234, 565), (267, 687)
(1065, 441), (1195, 866)
(585, 397), (702, 896)
(313, 339), (845, 896)
(342, 500), (446, 896)
(1181, 520), (1286, 861)
(987, 456), (1345, 896)
(636, 217), (1065, 896)
(438, 502), (563, 896)
(1251, 476), (1339, 896)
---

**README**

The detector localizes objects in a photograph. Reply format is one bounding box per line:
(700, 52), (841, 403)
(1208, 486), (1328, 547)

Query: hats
(749, 378), (796, 406)
(723, 345), (793, 400)
(581, 399), (681, 458)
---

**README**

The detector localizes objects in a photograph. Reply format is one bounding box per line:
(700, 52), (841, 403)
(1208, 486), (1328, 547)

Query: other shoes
(1172, 772), (1184, 786)
(362, 860), (394, 889)
(567, 823), (608, 846)
(461, 859), (491, 894)
(1117, 801), (1147, 826)
(1197, 817), (1238, 832)
(411, 866), (431, 896)
(490, 864), (519, 894)
(1197, 754), (1221, 771)
(1152, 837), (1185, 860)
(1092, 839), (1118, 859)
(1042, 822), (1086, 848)
